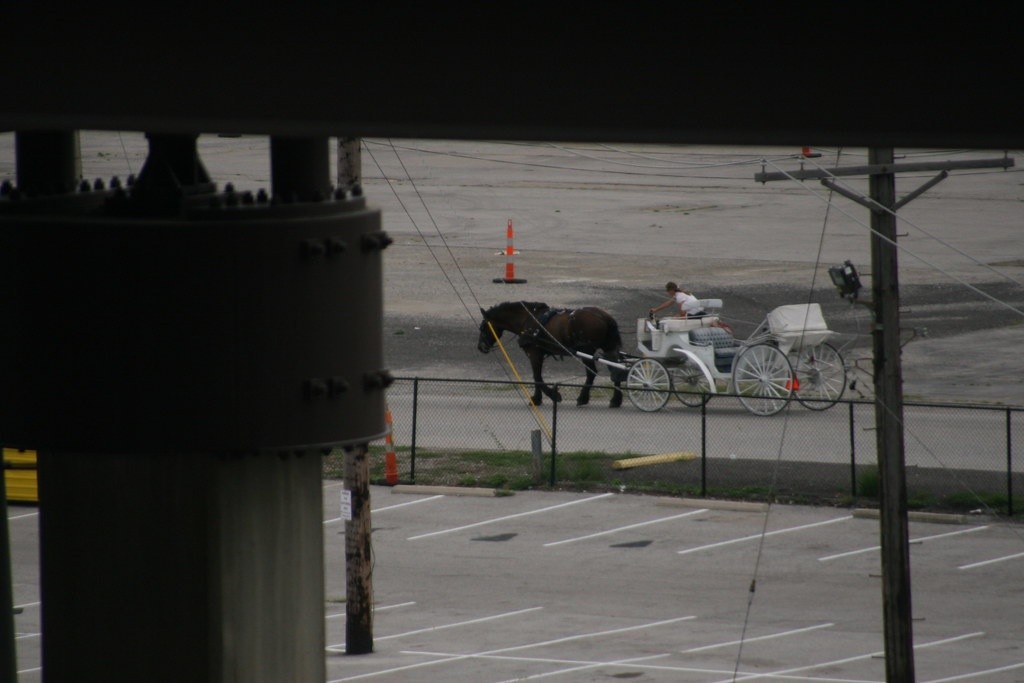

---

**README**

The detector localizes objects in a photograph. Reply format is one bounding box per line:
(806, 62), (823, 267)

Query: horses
(478, 300), (623, 408)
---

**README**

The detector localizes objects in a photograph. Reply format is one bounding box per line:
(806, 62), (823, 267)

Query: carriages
(478, 298), (848, 417)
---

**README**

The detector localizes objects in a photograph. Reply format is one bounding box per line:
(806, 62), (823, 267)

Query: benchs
(690, 327), (743, 368)
(646, 298), (723, 352)
(764, 302), (832, 354)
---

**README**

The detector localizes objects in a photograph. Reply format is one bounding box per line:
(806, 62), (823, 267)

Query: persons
(649, 282), (706, 320)
